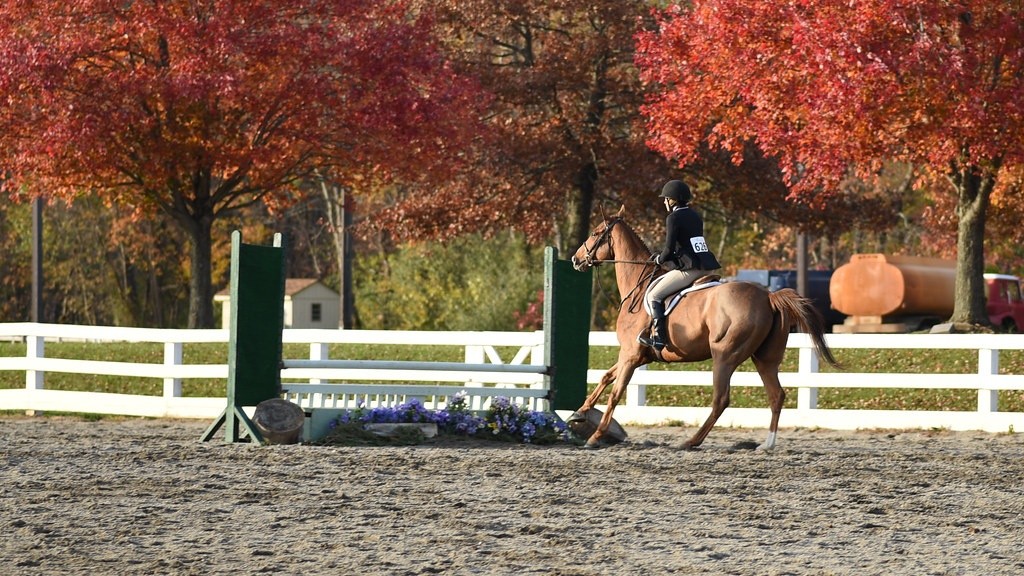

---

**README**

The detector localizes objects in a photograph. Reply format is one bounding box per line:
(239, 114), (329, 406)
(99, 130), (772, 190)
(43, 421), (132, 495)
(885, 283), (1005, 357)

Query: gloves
(654, 255), (660, 264)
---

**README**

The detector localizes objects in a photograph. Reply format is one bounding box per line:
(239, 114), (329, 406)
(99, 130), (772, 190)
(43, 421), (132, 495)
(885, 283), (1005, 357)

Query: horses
(566, 204), (846, 453)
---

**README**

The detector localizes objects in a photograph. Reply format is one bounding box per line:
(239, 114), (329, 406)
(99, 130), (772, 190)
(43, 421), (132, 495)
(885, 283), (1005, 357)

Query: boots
(639, 302), (670, 351)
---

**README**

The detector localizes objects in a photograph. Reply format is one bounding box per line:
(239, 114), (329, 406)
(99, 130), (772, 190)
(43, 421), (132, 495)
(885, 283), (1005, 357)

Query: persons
(639, 180), (721, 345)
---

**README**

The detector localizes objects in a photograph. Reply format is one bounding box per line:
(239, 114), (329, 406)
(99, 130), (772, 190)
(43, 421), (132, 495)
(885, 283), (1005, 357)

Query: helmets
(658, 180), (690, 203)
